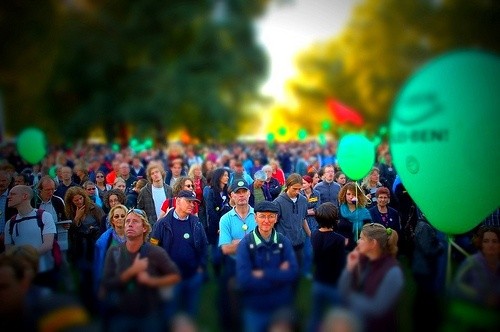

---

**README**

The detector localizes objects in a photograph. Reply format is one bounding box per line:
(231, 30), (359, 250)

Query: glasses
(88, 187), (96, 190)
(127, 208), (146, 219)
(184, 183), (194, 187)
(96, 175), (104, 177)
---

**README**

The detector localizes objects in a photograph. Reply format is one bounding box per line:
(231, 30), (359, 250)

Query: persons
(0, 137), (500, 332)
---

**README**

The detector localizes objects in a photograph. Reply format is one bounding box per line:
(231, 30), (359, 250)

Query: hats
(178, 190), (201, 204)
(226, 177), (248, 193)
(254, 200), (281, 214)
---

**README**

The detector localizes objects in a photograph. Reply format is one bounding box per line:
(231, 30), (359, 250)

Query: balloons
(387, 48), (500, 244)
(16, 126), (47, 164)
(336, 133), (376, 181)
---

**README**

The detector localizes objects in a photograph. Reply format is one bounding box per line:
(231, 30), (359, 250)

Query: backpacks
(9, 207), (62, 265)
